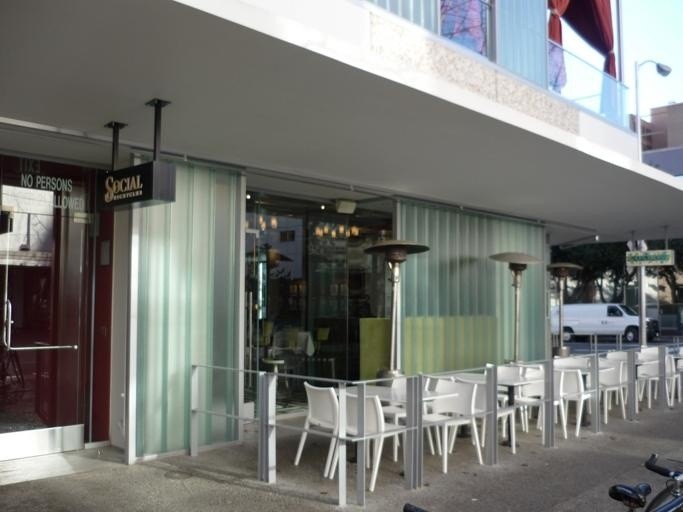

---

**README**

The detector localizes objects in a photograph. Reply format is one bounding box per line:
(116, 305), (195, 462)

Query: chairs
(423, 379), (483, 474)
(523, 369), (567, 440)
(294, 380), (370, 478)
(458, 378), (516, 456)
(382, 378), (434, 462)
(330, 390), (407, 492)
(554, 347), (682, 439)
(484, 363), (524, 437)
(427, 374), (475, 451)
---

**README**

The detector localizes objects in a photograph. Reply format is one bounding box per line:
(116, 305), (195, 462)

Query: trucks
(551, 302), (660, 342)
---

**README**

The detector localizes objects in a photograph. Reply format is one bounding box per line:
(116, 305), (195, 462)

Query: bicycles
(609, 453), (683, 512)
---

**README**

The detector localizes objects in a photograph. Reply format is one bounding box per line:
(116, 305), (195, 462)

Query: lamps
(489, 252), (545, 364)
(364, 241), (431, 382)
(547, 262), (585, 357)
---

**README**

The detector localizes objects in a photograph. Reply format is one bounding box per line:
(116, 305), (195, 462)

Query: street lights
(633, 57), (673, 161)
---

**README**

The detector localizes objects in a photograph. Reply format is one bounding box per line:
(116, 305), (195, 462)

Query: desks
(434, 372), (543, 448)
(329, 385), (459, 487)
